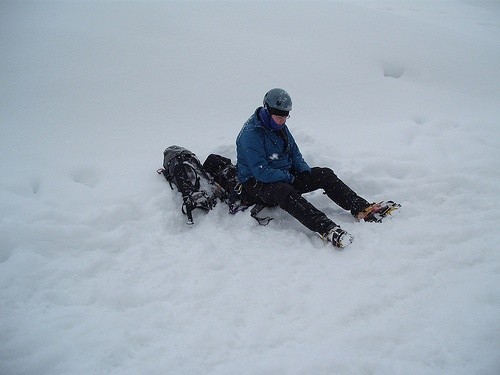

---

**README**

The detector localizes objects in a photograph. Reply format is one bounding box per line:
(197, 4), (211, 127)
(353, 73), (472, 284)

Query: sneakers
(356, 198), (401, 226)
(330, 229), (352, 249)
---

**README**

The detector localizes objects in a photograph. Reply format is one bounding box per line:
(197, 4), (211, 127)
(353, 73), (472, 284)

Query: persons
(235, 88), (401, 248)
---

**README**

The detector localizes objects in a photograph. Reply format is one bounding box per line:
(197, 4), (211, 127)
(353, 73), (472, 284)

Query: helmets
(263, 87), (294, 116)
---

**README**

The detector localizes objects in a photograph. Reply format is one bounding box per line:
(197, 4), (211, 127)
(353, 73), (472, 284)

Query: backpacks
(203, 151), (250, 206)
(162, 143), (228, 227)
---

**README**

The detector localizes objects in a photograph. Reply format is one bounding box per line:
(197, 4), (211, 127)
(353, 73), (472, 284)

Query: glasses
(272, 114), (291, 120)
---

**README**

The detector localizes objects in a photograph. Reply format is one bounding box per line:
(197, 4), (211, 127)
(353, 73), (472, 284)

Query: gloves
(294, 169), (319, 192)
